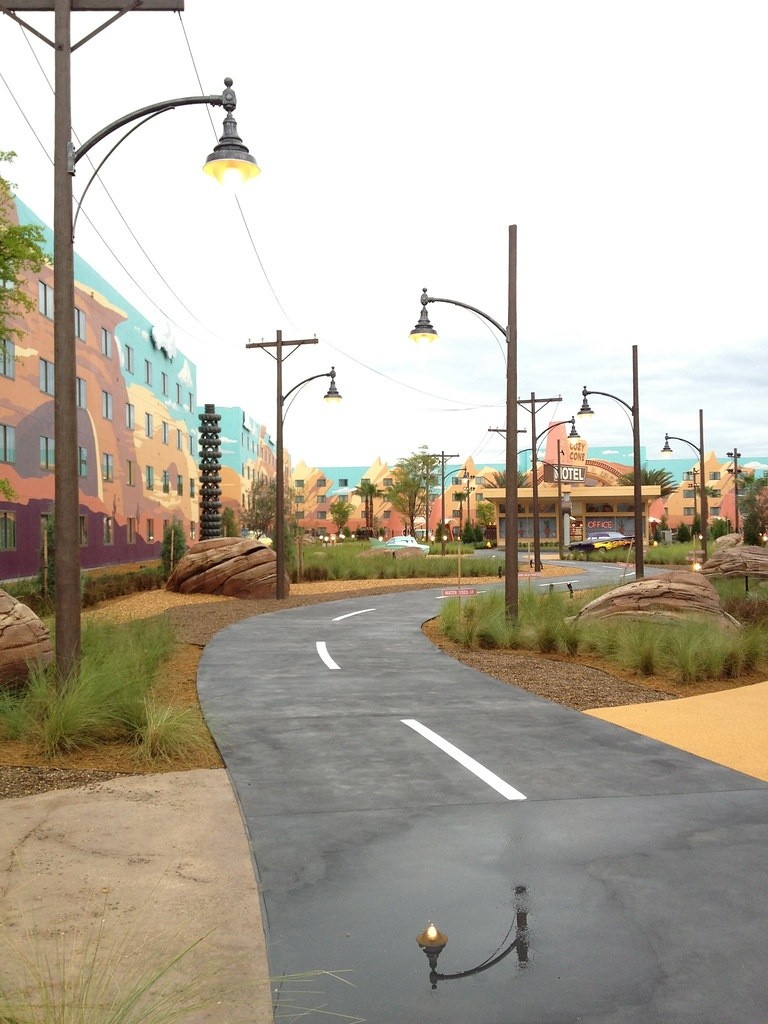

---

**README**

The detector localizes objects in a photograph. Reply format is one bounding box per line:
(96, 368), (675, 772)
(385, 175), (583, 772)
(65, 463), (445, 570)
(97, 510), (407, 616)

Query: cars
(587, 531), (624, 540)
(387, 536), (417, 545)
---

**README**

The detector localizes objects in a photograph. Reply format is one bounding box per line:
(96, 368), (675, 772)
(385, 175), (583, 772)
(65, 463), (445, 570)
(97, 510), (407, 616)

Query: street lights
(463, 471), (476, 522)
(531, 416), (580, 571)
(55, 79), (260, 677)
(276, 367), (343, 599)
(408, 287), (518, 622)
(687, 467), (699, 515)
(530, 458), (563, 553)
(441, 467), (469, 556)
(726, 448), (742, 533)
(661, 433), (708, 563)
(576, 385), (643, 579)
